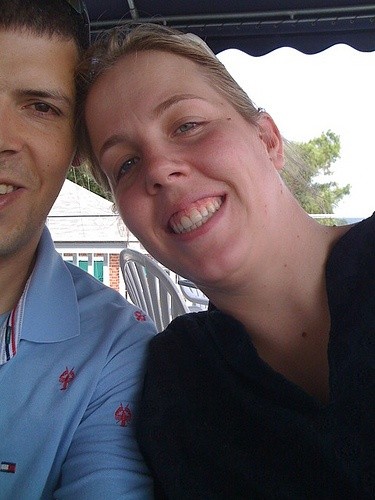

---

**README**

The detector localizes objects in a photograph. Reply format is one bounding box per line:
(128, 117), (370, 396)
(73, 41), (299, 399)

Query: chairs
(120, 248), (212, 334)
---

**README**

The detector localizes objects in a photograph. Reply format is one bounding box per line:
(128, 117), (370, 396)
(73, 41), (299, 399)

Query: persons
(0, 0), (157, 500)
(76, 24), (375, 500)
